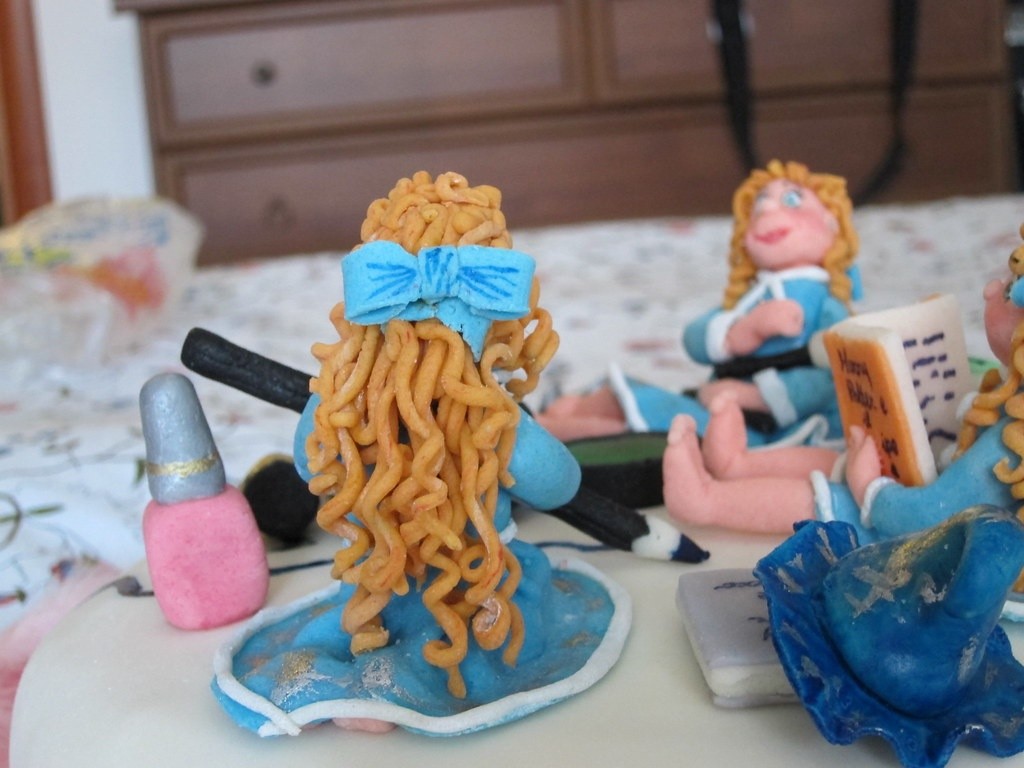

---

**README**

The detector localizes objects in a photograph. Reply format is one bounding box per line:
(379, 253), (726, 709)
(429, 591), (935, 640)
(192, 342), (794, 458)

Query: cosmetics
(140, 372), (269, 630)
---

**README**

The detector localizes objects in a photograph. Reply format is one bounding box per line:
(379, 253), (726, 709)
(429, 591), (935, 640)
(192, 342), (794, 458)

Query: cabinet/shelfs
(113, 0), (1017, 266)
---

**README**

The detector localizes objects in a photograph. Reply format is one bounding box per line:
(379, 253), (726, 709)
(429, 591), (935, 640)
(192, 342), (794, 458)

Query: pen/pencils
(176, 325), (711, 566)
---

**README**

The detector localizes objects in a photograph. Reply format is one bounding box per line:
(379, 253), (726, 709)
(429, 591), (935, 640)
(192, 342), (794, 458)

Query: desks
(8, 194), (1024, 768)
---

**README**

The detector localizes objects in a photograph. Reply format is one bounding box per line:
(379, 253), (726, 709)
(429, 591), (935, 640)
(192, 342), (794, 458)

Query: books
(824, 294), (973, 487)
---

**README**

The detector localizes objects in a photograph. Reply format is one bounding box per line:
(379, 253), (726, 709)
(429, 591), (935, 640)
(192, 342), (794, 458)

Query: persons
(213, 171), (633, 737)
(535, 162), (860, 447)
(665, 223), (1024, 544)
